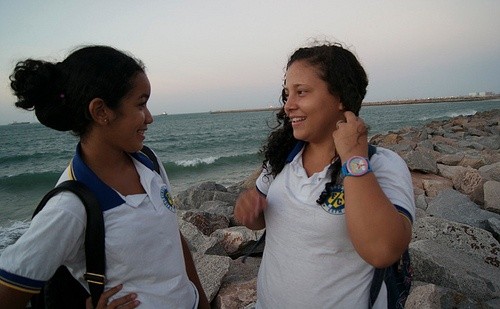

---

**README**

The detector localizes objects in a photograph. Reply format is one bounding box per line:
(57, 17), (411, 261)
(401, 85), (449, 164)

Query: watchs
(341, 156), (372, 177)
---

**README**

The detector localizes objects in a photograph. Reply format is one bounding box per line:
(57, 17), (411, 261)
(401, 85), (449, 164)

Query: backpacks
(30, 147), (161, 309)
(292, 139), (412, 309)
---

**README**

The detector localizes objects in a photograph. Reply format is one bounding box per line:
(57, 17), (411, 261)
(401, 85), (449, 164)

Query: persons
(0, 44), (209, 309)
(233, 44), (415, 309)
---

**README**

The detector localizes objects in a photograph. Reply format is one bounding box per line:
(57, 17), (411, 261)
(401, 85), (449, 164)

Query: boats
(11, 120), (30, 125)
